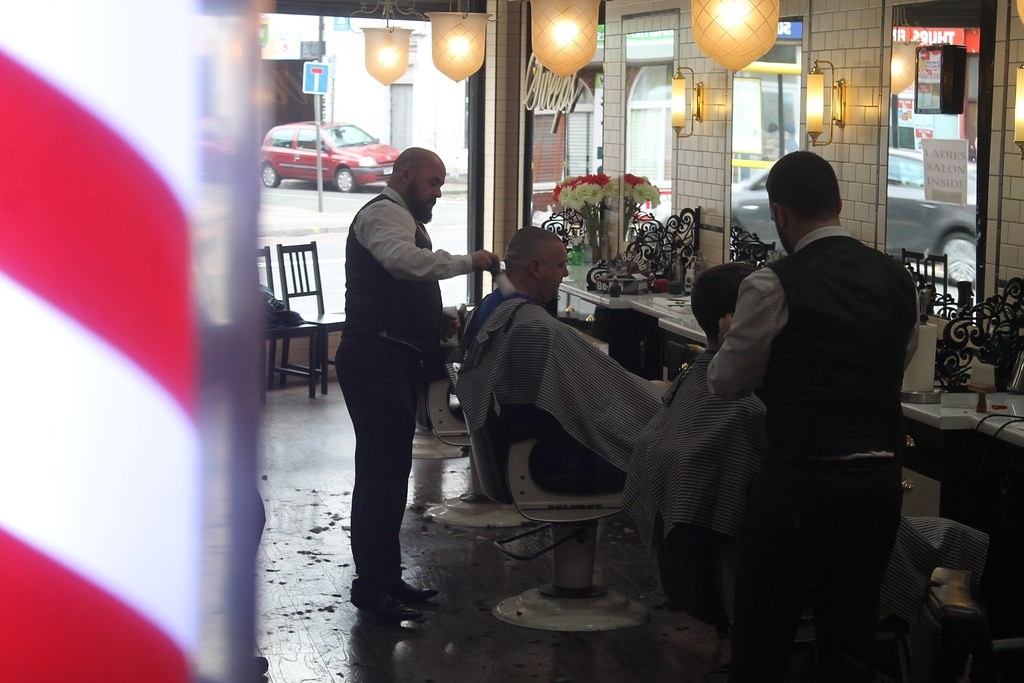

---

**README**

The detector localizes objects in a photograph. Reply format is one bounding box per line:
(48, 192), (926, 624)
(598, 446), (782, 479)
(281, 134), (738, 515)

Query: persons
(460, 226), (670, 497)
(334, 146), (501, 618)
(692, 262), (989, 632)
(706, 150), (919, 683)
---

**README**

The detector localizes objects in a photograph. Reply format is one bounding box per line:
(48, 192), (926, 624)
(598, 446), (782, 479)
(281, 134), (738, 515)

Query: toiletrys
(568, 243), (583, 266)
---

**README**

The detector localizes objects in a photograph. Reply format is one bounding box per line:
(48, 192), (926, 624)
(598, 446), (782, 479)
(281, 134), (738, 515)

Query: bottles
(571, 244), (582, 266)
(672, 257), (683, 280)
(685, 268), (696, 292)
(610, 280), (620, 297)
(696, 258), (705, 275)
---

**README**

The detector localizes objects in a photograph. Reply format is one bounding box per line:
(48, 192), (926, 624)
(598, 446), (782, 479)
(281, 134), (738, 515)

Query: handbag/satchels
(261, 284), (304, 328)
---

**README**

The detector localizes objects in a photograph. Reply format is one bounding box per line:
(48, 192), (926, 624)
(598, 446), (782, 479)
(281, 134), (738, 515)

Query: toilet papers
(902, 323), (938, 392)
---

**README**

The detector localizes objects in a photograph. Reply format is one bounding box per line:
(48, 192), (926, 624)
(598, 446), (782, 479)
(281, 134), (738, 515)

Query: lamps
(530, 0), (601, 78)
(672, 65), (704, 138)
(348, 1), (515, 83)
(890, 5), (922, 96)
(806, 59), (846, 146)
(691, 0), (780, 74)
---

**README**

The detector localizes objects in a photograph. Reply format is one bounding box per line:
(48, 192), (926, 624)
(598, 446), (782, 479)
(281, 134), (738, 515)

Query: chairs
(445, 360), (651, 632)
(250, 241), (347, 399)
(631, 432), (977, 683)
(416, 304), (541, 530)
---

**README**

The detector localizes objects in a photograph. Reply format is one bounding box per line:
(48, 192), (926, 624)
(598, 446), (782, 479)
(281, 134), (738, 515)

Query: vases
(624, 213), (631, 239)
(582, 218), (600, 262)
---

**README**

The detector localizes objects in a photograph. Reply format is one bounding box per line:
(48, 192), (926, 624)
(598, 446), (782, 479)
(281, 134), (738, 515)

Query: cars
(260, 121), (401, 193)
(628, 147), (978, 288)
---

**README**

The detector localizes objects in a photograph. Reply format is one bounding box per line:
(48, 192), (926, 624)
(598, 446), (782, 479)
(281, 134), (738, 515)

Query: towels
(462, 286), (539, 351)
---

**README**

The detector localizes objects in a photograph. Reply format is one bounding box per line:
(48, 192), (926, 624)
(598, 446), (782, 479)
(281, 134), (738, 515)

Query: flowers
(625, 174), (661, 220)
(552, 174), (610, 234)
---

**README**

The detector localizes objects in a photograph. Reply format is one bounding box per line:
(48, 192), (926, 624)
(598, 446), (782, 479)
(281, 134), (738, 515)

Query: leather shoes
(393, 578), (439, 603)
(351, 585), (422, 619)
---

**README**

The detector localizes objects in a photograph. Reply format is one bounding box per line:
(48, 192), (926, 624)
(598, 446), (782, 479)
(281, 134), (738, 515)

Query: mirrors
(730, 15), (802, 265)
(624, 28), (677, 241)
(884, 0), (997, 327)
